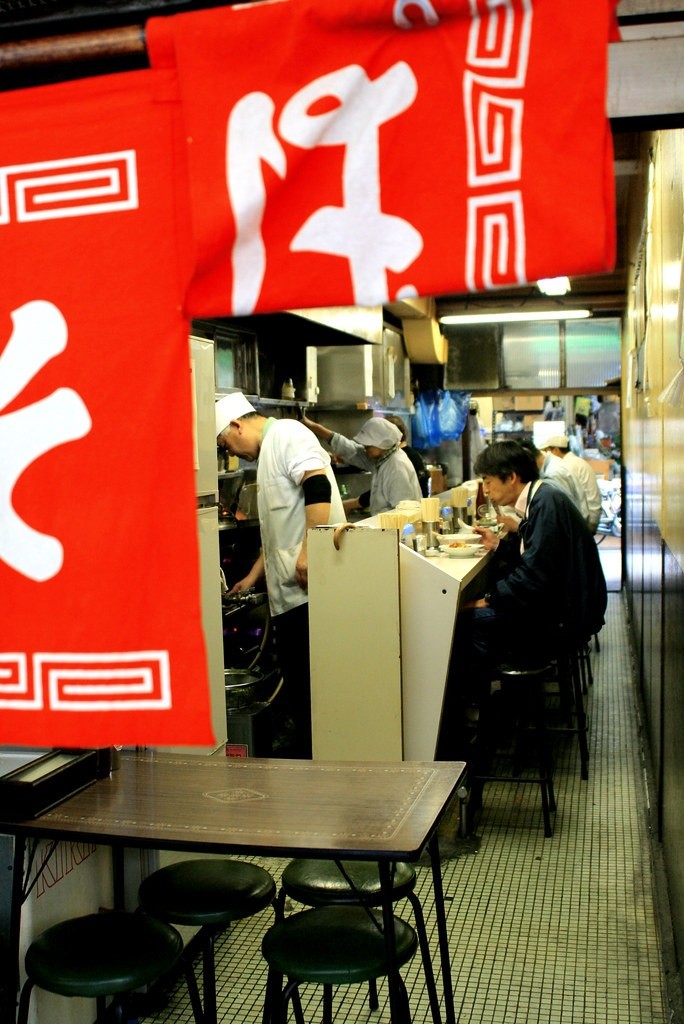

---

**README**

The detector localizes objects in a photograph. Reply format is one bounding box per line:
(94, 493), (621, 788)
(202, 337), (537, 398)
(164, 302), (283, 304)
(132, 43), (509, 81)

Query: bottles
(475, 483), (488, 520)
(401, 524), (416, 549)
(340, 484), (352, 500)
(442, 506), (454, 534)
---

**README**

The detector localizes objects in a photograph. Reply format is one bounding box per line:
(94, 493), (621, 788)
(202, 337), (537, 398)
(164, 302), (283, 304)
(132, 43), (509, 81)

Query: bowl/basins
(438, 543), (485, 558)
(436, 534), (482, 544)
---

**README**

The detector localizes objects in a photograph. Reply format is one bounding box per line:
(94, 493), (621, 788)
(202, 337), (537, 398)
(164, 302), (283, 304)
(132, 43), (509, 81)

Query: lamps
(437, 304), (593, 324)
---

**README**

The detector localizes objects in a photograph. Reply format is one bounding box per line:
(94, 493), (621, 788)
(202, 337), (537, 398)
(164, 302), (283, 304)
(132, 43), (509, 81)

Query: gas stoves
(222, 583), (268, 625)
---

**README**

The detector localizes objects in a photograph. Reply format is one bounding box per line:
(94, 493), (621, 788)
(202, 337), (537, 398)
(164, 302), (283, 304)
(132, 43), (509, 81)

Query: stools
(18, 858), (441, 1024)
(468, 632), (599, 839)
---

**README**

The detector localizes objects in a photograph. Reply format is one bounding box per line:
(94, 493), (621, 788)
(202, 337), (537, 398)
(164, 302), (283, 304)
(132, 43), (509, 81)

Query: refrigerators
(122, 335), (228, 756)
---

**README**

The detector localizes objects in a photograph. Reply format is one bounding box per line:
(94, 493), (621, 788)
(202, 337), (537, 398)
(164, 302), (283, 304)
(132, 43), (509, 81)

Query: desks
(1, 753), (469, 1023)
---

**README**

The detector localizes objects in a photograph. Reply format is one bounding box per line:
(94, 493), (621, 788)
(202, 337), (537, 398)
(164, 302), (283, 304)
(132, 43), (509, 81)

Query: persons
(217, 386), (349, 760)
(296, 406), (427, 517)
(595, 395), (621, 451)
(452, 412), (608, 776)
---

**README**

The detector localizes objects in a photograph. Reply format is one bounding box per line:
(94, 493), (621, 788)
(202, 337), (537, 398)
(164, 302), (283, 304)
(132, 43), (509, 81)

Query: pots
(222, 594), (257, 606)
(224, 668), (264, 708)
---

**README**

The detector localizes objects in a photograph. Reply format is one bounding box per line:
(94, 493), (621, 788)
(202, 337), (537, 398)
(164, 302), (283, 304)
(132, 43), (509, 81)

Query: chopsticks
(452, 487), (468, 507)
(379, 513), (407, 530)
(420, 498), (440, 522)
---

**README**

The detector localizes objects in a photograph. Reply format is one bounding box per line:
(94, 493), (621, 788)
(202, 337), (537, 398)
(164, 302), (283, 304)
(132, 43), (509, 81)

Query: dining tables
(307, 480), (524, 761)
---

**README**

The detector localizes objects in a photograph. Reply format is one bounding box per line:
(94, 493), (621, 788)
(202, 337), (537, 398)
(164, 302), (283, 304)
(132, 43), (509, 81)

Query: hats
(353, 418), (403, 451)
(215, 392), (256, 438)
(532, 420), (569, 450)
